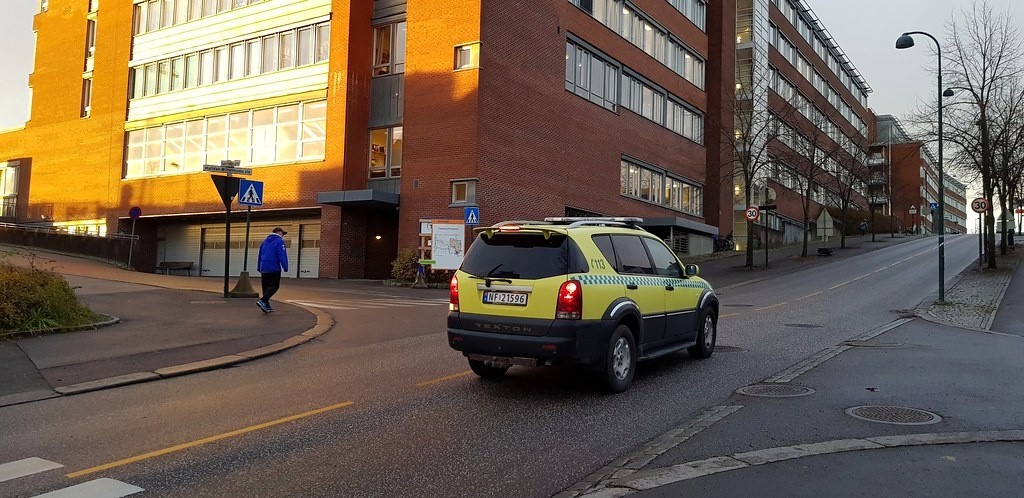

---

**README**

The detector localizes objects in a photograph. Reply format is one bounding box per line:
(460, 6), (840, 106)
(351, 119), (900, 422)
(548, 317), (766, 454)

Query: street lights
(942, 86), (989, 264)
(896, 31), (943, 306)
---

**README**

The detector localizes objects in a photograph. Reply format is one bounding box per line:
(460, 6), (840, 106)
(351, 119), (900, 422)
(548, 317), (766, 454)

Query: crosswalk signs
(462, 208), (481, 224)
(238, 179), (264, 206)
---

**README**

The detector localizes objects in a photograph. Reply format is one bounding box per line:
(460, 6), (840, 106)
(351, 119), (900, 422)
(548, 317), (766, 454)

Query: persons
(726, 229), (734, 241)
(256, 226), (288, 313)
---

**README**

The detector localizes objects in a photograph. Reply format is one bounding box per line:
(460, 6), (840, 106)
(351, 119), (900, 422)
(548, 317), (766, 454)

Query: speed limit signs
(745, 207), (759, 221)
(972, 198), (989, 213)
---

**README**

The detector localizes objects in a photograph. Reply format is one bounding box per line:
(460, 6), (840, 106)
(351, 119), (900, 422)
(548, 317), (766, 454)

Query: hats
(273, 228), (287, 235)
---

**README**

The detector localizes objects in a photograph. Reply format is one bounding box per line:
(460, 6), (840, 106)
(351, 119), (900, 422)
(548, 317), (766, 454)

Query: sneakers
(256, 300), (268, 313)
(266, 307), (275, 312)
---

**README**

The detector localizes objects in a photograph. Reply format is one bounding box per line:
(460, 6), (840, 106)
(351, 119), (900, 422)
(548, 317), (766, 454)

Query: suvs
(446, 213), (719, 391)
